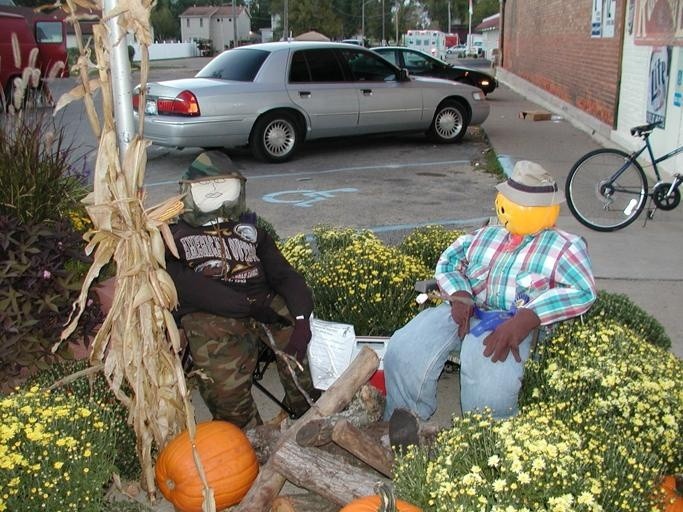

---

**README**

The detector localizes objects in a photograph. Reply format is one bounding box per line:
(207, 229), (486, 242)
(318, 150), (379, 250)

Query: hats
(492, 160), (568, 208)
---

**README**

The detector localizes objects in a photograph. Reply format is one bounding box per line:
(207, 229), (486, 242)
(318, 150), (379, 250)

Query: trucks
(407, 30), (446, 65)
(1, 4), (70, 113)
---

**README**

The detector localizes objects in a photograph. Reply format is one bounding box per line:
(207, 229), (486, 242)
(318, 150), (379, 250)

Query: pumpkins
(650, 473), (683, 512)
(340, 484), (420, 512)
(156, 419), (257, 512)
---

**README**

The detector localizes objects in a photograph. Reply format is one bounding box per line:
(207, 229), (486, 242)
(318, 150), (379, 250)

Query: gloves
(448, 290), (475, 340)
(248, 301), (292, 327)
(483, 307), (541, 363)
(284, 318), (311, 364)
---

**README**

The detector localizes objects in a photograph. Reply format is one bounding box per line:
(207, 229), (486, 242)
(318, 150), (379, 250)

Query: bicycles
(565, 120), (683, 232)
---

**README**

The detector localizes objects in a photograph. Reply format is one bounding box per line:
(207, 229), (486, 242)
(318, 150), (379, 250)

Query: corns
(156, 201), (184, 221)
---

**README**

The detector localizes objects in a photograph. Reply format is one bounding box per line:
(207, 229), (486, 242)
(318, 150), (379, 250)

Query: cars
(370, 47), (498, 96)
(446, 46), (466, 54)
(133, 40), (490, 164)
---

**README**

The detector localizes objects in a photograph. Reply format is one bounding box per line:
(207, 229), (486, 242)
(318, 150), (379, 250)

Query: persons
(154, 149), (314, 426)
(383, 161), (596, 433)
